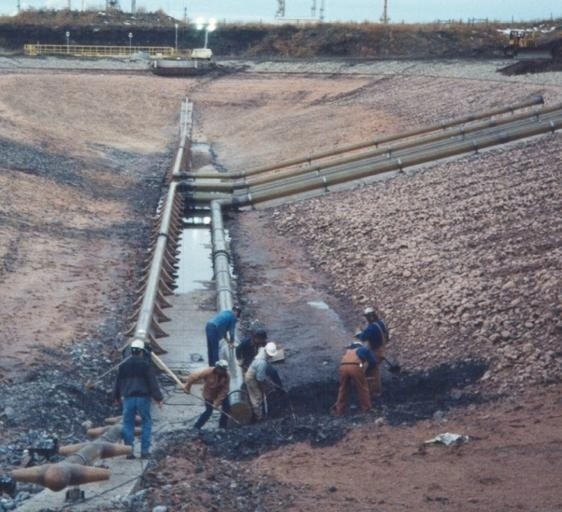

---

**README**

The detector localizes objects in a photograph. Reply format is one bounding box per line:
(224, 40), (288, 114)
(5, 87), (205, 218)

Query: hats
(215, 359), (229, 369)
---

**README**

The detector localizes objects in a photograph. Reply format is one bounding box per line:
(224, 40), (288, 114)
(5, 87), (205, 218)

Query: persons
(183, 359), (231, 430)
(245, 340), (279, 420)
(235, 329), (266, 371)
(113, 339), (163, 460)
(205, 305), (242, 366)
(335, 340), (377, 413)
(355, 307), (389, 393)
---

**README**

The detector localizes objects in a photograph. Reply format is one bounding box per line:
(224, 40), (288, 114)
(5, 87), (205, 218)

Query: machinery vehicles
(506, 29), (542, 52)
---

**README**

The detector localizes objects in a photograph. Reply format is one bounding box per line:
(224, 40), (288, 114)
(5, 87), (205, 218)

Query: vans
(191, 48), (214, 60)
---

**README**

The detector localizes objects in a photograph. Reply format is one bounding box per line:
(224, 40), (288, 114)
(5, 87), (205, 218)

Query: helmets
(364, 307), (375, 314)
(265, 342), (277, 356)
(131, 340), (144, 350)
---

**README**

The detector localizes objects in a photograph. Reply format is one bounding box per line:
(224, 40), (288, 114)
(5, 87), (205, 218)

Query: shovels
(384, 359), (401, 373)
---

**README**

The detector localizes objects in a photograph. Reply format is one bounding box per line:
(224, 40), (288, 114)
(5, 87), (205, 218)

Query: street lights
(127, 32), (133, 57)
(173, 23), (179, 54)
(66, 31), (71, 54)
